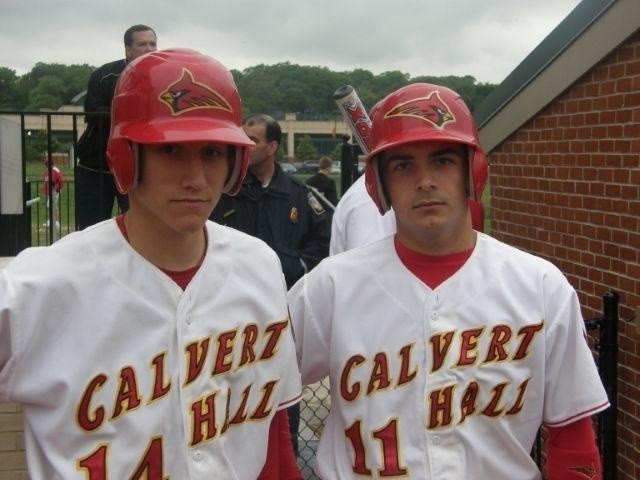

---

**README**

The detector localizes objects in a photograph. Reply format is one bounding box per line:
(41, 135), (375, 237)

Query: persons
(328, 168), (393, 258)
(206, 111), (329, 290)
(302, 156), (339, 206)
(0, 49), (309, 480)
(42, 159), (63, 226)
(292, 83), (604, 478)
(74, 23), (156, 235)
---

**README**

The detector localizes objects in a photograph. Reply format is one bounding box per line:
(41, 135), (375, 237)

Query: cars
(279, 158), (367, 178)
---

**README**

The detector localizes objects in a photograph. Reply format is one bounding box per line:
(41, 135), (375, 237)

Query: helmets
(106, 48), (256, 196)
(365, 82), (488, 215)
